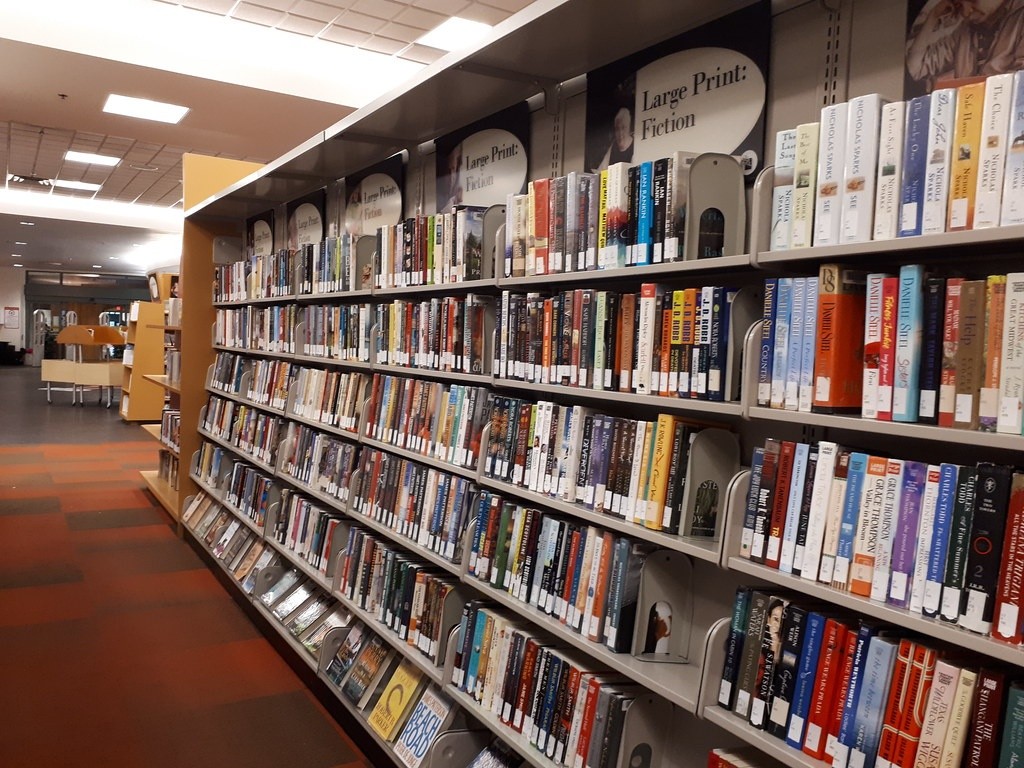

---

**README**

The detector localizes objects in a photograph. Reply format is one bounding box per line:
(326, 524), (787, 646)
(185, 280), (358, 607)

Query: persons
(592, 108), (634, 174)
(247, 231), (254, 260)
(348, 187), (362, 237)
(907, 0), (1024, 90)
(436, 148), (463, 214)
(286, 214), (298, 250)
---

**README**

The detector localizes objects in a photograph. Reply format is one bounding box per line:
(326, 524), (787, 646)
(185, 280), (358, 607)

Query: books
(212, 348), (1024, 650)
(120, 297), (187, 491)
(206, 263), (1021, 435)
(178, 394), (1024, 767)
(207, 71), (1024, 310)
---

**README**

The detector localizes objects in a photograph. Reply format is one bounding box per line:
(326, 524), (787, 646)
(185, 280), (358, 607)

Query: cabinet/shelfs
(41, 0), (1024, 768)
